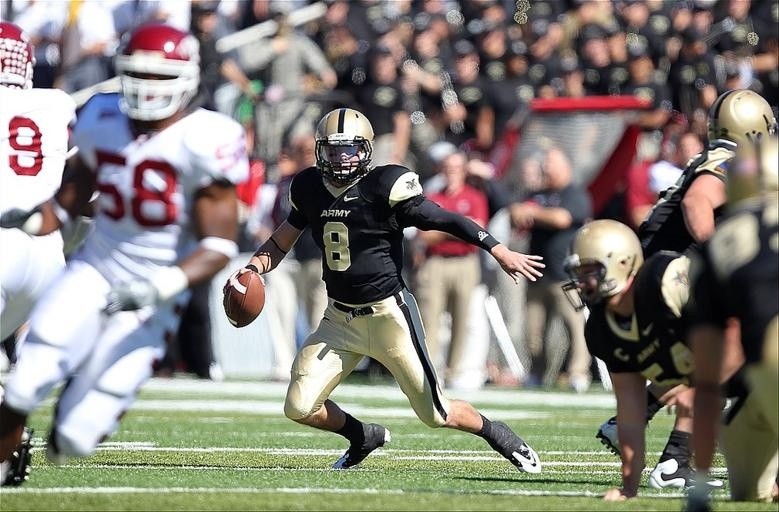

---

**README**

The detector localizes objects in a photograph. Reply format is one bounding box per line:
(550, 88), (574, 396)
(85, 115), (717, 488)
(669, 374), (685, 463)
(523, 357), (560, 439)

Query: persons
(1, 24), (77, 488)
(0, 0), (779, 397)
(222, 109), (545, 474)
(0, 24), (249, 466)
(560, 87), (778, 512)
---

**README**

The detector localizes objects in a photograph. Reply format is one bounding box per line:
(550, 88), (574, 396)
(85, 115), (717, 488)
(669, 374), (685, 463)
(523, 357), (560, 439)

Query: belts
(333, 301), (374, 323)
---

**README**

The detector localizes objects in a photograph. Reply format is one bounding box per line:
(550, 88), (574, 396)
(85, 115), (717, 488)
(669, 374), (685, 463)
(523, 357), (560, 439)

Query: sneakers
(595, 417), (622, 460)
(487, 421), (542, 474)
(5, 426), (36, 487)
(331, 424), (391, 470)
(647, 456), (726, 491)
(46, 375), (71, 467)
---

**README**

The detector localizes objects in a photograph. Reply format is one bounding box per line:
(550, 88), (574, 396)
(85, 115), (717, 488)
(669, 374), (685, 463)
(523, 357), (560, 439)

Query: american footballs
(223, 270), (265, 328)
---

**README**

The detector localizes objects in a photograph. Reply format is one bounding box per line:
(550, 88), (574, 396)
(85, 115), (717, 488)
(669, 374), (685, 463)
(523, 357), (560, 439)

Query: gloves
(1, 206), (42, 229)
(102, 279), (160, 317)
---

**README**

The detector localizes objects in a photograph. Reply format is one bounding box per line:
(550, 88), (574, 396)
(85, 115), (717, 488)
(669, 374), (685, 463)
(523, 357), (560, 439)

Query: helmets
(561, 219), (645, 312)
(0, 22), (35, 90)
(114, 26), (201, 122)
(705, 88), (777, 148)
(314, 108), (376, 188)
